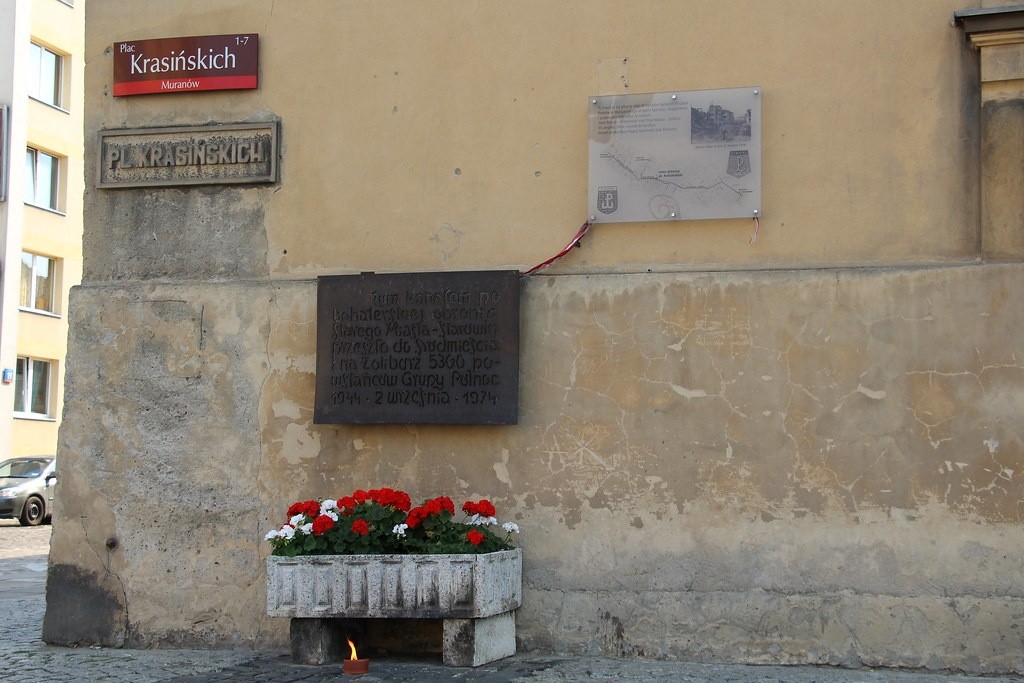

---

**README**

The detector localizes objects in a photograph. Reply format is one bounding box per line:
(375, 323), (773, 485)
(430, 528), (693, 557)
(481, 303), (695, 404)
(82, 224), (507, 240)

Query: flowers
(262, 488), (520, 555)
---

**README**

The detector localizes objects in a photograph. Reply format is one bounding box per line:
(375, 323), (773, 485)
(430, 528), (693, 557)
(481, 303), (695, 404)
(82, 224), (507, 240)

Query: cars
(0, 455), (57, 525)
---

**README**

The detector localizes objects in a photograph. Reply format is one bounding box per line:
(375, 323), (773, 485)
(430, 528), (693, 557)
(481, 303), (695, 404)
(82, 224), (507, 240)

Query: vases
(267, 547), (523, 668)
